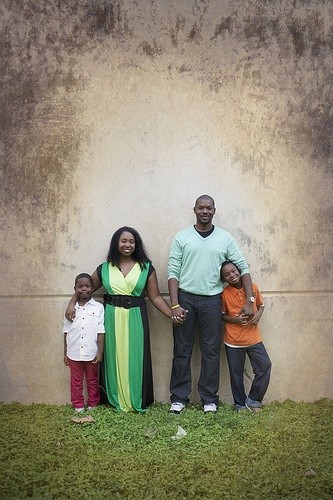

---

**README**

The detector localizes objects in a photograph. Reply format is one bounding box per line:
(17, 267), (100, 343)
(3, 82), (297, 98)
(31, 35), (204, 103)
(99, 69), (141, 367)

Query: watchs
(246, 297), (254, 302)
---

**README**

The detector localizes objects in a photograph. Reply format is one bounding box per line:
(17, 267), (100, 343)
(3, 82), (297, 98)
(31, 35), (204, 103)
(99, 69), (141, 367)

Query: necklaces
(120, 267), (127, 272)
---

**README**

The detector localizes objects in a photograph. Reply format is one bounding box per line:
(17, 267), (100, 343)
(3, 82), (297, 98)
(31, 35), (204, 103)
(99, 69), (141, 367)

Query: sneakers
(245, 396), (262, 412)
(235, 405), (246, 413)
(169, 402), (185, 411)
(204, 403), (217, 412)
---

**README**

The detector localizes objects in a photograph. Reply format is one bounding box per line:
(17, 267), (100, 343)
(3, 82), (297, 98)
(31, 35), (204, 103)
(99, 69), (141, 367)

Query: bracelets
(171, 304), (180, 309)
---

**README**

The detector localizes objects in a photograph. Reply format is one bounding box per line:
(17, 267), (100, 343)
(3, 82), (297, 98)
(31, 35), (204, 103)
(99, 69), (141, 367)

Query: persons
(65, 226), (188, 412)
(168, 194), (254, 414)
(62, 273), (105, 412)
(220, 260), (272, 414)
